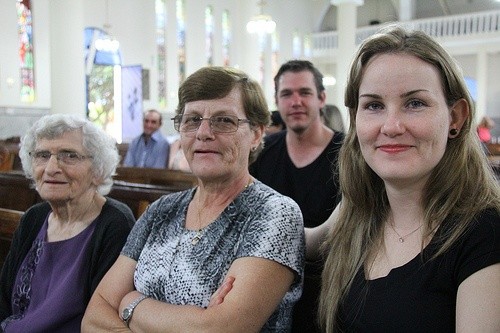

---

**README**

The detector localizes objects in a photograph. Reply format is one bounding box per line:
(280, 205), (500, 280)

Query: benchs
(0, 139), (201, 270)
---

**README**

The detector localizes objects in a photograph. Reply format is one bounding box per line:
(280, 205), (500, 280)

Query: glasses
(170, 114), (251, 134)
(27, 150), (95, 165)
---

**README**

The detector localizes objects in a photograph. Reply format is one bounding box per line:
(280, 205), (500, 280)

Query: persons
(246, 60), (347, 229)
(0, 113), (136, 333)
(81, 65), (305, 333)
(295, 25), (500, 333)
(124, 95), (494, 175)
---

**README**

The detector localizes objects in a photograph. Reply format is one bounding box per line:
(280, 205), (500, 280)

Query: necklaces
(388, 215), (424, 243)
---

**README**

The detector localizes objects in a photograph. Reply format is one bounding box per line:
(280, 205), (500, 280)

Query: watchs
(121, 294), (149, 329)
